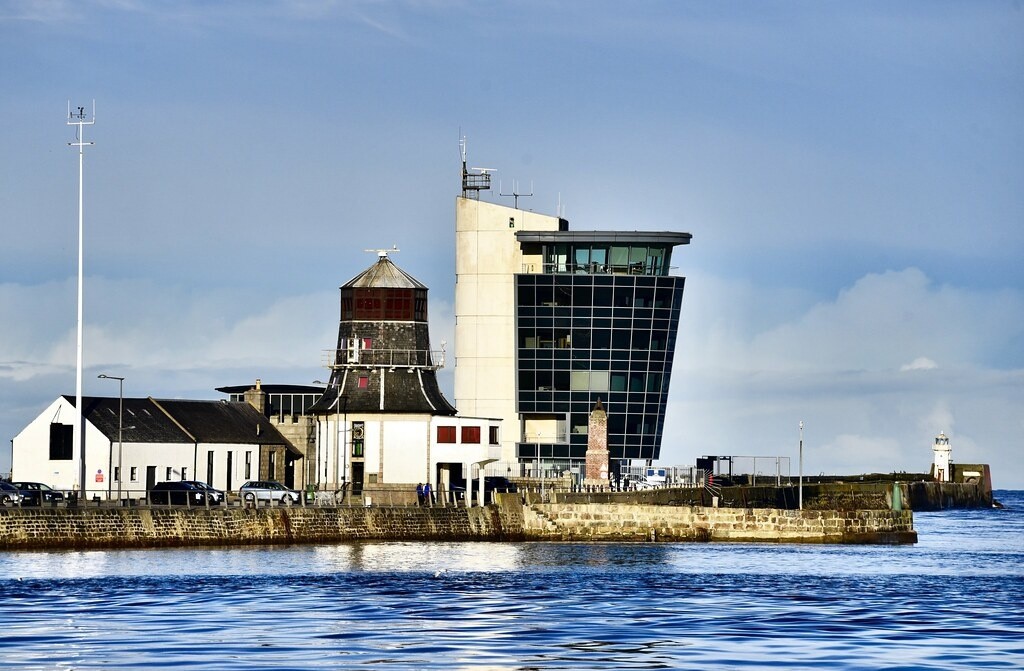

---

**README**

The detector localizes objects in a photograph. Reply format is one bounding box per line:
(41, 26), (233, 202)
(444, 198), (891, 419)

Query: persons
(624, 474), (630, 492)
(423, 482), (430, 505)
(610, 471), (617, 492)
(416, 482), (424, 506)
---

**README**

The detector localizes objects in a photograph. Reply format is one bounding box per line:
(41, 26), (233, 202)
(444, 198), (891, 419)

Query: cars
(1, 481), (64, 505)
(450, 477), (520, 501)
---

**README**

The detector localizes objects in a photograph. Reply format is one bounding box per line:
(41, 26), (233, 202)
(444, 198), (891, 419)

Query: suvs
(148, 479), (226, 506)
(238, 480), (302, 504)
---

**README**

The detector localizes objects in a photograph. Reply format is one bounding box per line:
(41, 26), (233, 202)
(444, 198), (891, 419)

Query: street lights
(799, 420), (803, 509)
(97, 373), (126, 511)
